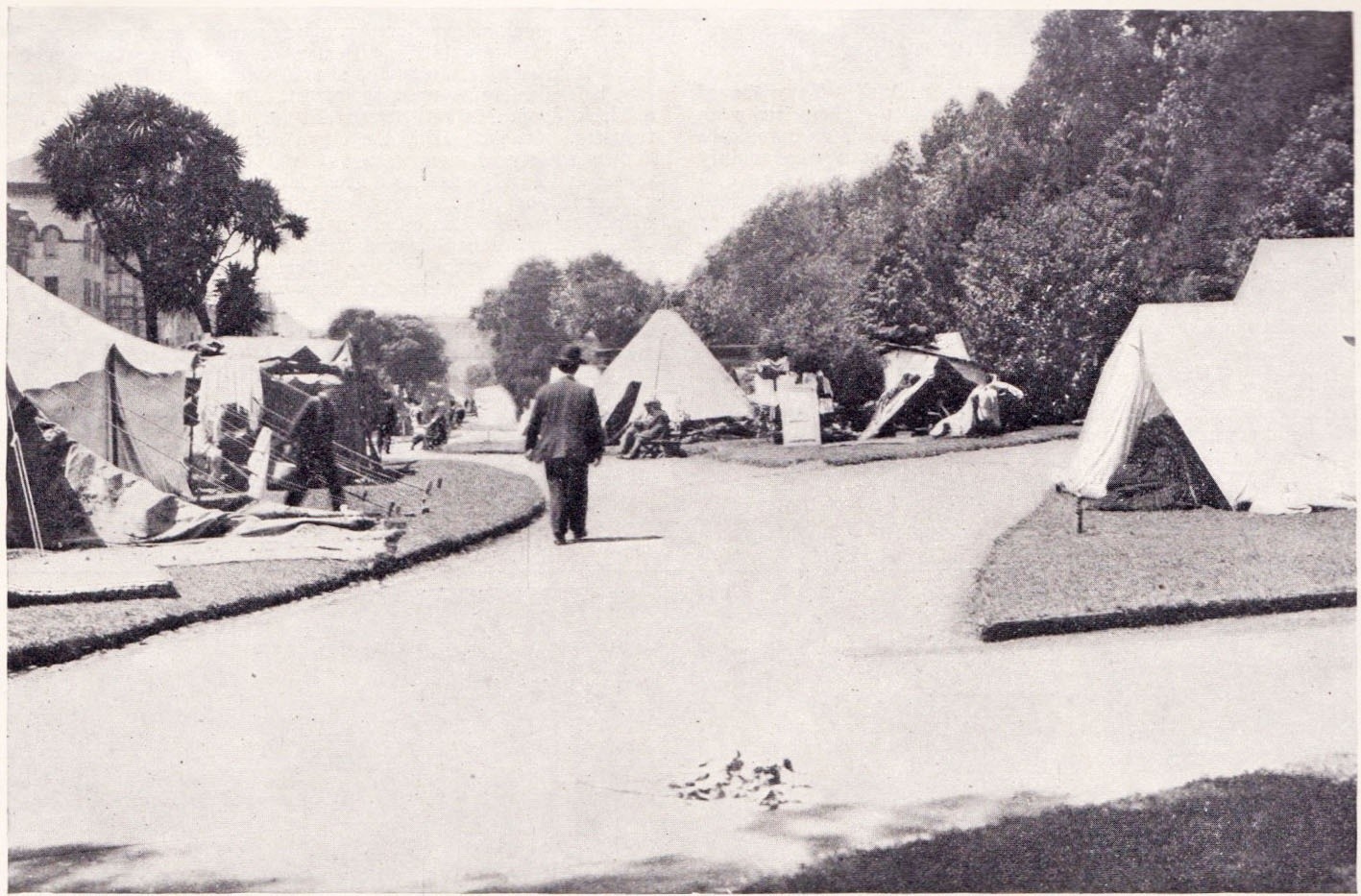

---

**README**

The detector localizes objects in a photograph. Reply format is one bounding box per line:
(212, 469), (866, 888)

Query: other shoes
(554, 538), (567, 545)
(574, 530), (588, 538)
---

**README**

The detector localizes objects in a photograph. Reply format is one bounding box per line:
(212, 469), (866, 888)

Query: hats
(550, 345), (587, 366)
(319, 373), (343, 386)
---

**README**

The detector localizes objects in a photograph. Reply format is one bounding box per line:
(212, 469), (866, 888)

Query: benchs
(638, 421), (684, 458)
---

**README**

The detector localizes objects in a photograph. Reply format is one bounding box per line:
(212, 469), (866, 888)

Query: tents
(8, 269), (365, 500)
(1063, 238), (1358, 513)
(552, 308), (754, 435)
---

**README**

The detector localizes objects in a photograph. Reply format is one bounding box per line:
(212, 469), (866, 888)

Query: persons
(373, 390), (478, 453)
(280, 382), (349, 511)
(523, 344), (606, 546)
(617, 396), (669, 460)
